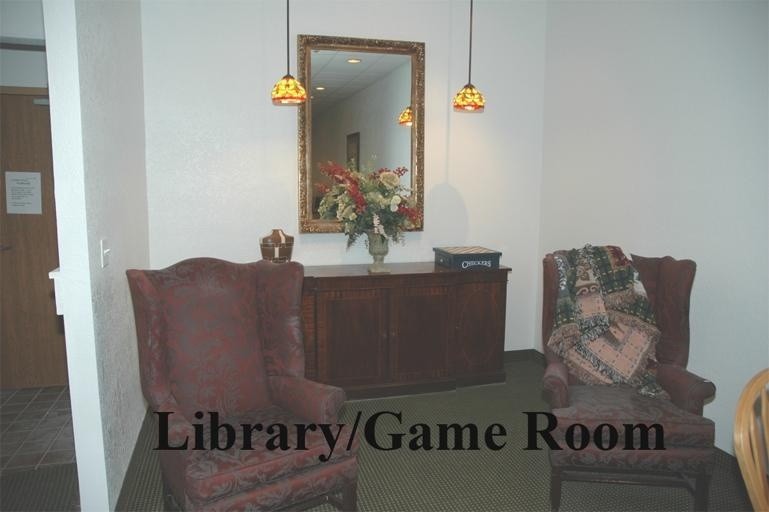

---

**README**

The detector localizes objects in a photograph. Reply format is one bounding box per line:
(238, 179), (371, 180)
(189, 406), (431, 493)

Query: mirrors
(295, 33), (425, 234)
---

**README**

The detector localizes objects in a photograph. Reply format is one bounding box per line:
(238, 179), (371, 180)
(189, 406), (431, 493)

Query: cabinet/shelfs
(300, 263), (512, 402)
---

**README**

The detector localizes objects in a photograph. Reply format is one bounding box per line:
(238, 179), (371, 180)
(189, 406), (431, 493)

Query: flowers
(319, 158), (423, 251)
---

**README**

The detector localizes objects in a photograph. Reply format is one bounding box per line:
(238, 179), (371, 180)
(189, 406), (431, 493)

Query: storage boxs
(433, 246), (502, 272)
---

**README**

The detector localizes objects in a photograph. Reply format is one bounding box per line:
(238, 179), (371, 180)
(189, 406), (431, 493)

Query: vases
(365, 233), (394, 274)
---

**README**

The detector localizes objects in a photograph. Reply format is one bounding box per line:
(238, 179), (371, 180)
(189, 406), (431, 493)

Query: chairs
(732, 367), (769, 512)
(539, 247), (717, 512)
(123, 256), (360, 512)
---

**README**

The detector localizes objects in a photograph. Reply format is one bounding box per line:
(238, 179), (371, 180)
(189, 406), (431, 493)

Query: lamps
(272, 0), (308, 107)
(399, 105), (413, 127)
(452, 0), (485, 111)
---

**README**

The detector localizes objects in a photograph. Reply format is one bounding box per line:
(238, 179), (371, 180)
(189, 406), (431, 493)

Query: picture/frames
(344, 132), (360, 173)
